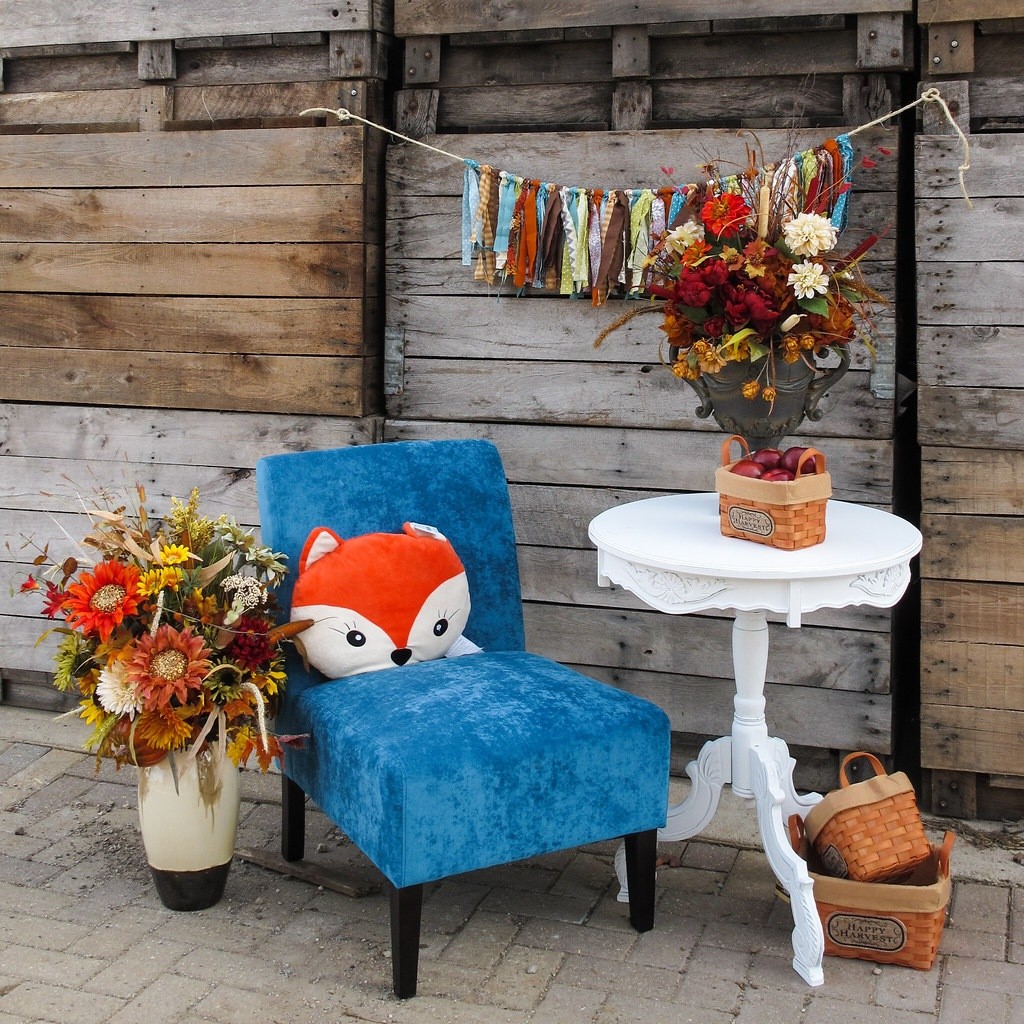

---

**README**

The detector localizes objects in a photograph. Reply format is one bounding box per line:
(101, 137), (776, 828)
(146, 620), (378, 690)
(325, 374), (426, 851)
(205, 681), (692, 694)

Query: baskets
(714, 435), (831, 549)
(804, 751), (930, 882)
(789, 813), (955, 970)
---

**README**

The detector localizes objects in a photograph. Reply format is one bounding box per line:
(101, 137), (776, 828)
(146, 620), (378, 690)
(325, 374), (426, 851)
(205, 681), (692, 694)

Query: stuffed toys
(290, 523), (472, 679)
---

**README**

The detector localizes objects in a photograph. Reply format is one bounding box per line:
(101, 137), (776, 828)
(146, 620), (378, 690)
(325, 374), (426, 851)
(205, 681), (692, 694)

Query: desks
(588, 492), (923, 986)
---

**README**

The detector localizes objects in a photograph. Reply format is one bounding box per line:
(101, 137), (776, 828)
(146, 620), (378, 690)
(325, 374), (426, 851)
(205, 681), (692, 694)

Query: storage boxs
(773, 815), (954, 972)
(714, 436), (833, 551)
(803, 752), (931, 884)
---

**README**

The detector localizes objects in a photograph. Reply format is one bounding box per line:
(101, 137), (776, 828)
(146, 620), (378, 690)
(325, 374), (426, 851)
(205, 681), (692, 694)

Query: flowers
(593, 59), (894, 413)
(9, 478), (313, 826)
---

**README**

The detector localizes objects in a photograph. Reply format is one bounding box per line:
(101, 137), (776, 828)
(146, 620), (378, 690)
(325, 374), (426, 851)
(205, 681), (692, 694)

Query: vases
(138, 740), (240, 912)
(662, 329), (851, 460)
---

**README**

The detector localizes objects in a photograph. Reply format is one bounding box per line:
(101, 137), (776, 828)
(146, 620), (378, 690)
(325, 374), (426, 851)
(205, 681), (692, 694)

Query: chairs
(255, 438), (671, 998)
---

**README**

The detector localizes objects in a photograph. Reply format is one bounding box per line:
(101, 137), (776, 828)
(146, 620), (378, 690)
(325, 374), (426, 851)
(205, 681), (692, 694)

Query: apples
(729, 446), (816, 481)
